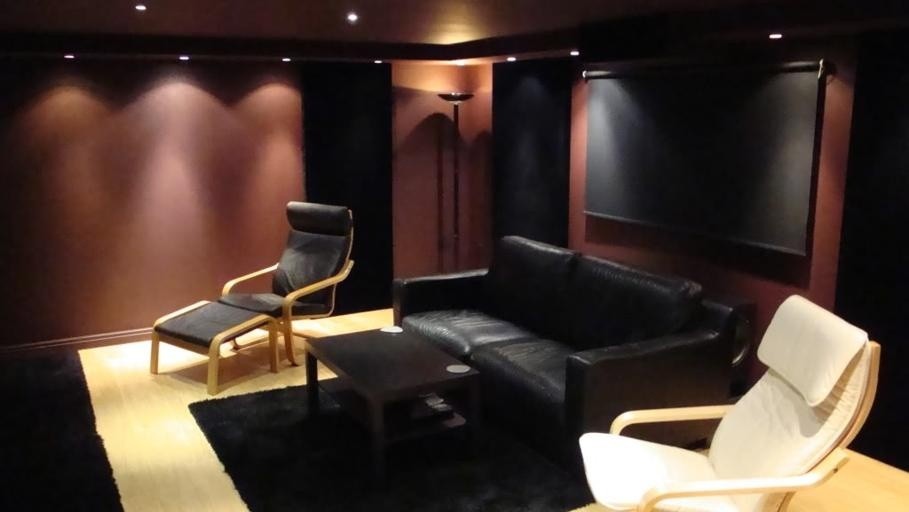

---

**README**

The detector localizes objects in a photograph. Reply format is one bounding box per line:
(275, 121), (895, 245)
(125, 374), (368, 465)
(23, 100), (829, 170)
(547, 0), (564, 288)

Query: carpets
(1, 343), (123, 512)
(189, 374), (599, 512)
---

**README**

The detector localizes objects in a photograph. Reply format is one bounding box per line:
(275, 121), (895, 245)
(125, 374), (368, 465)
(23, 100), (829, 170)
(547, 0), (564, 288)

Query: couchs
(393, 232), (761, 479)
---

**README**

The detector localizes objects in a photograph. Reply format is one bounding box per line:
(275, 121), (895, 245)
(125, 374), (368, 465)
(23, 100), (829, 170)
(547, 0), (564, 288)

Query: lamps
(438, 89), (476, 271)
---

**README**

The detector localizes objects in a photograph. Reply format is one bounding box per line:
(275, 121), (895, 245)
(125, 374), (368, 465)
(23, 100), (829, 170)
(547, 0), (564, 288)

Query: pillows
(488, 235), (581, 323)
(565, 255), (704, 346)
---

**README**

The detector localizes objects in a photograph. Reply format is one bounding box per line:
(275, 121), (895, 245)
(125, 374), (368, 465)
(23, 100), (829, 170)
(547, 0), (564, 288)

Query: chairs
(149, 199), (357, 395)
(578, 293), (881, 510)
